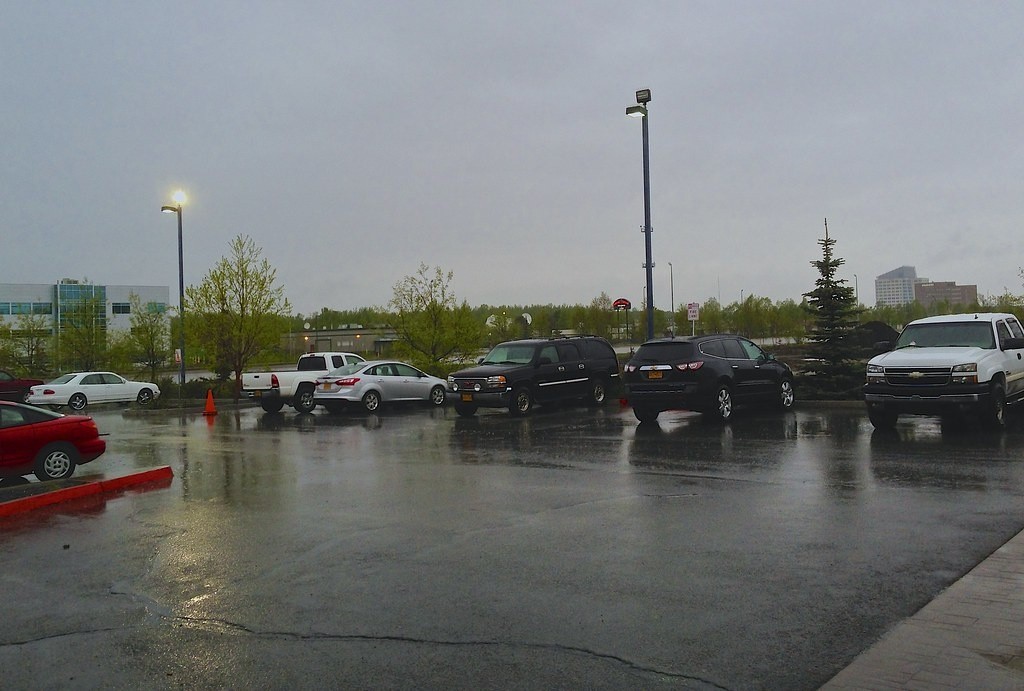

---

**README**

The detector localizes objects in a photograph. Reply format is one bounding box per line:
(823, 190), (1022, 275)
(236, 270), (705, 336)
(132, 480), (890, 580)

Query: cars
(28, 371), (161, 410)
(0, 370), (45, 403)
(312, 361), (449, 416)
(0, 401), (106, 483)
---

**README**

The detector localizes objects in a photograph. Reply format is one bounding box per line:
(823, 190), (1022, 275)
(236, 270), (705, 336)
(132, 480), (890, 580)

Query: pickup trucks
(241, 352), (386, 416)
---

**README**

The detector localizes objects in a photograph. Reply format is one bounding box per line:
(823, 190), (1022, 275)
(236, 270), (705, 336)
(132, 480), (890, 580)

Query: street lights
(741, 289), (744, 304)
(160, 189), (187, 400)
(643, 286), (647, 342)
(668, 262), (674, 338)
(853, 274), (858, 307)
(625, 89), (655, 342)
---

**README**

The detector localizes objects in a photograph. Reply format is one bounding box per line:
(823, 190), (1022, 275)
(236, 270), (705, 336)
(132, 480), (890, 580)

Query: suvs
(863, 312), (1024, 433)
(445, 333), (620, 418)
(622, 332), (797, 422)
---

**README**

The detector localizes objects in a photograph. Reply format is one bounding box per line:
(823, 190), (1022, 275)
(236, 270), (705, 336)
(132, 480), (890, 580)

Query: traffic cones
(203, 390), (219, 417)
